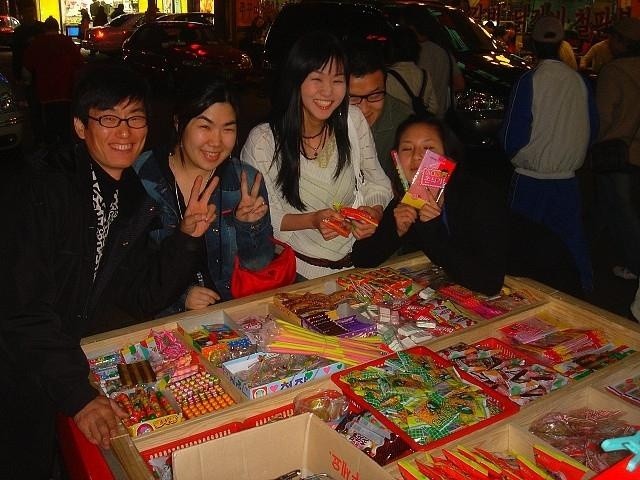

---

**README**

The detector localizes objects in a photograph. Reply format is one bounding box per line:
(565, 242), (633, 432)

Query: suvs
(264, 0), (601, 152)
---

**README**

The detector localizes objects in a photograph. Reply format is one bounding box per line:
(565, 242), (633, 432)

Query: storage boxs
(172, 410), (394, 479)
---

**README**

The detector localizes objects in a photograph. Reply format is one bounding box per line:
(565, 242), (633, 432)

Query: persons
(0, 0), (640, 320)
(0, 73), (222, 480)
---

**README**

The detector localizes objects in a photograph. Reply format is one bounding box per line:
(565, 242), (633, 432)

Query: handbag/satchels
(444, 106), (461, 132)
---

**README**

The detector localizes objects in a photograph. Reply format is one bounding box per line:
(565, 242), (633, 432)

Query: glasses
(348, 79), (387, 106)
(85, 113), (149, 129)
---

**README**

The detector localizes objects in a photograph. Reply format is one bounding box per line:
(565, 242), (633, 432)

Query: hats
(531, 16), (565, 44)
(600, 15), (640, 42)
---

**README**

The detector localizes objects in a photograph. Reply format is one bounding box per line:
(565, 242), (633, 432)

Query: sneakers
(611, 264), (638, 281)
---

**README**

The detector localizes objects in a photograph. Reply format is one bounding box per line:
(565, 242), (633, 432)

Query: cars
(122, 20), (254, 84)
(84, 13), (166, 55)
(0, 73), (24, 153)
(157, 12), (215, 35)
